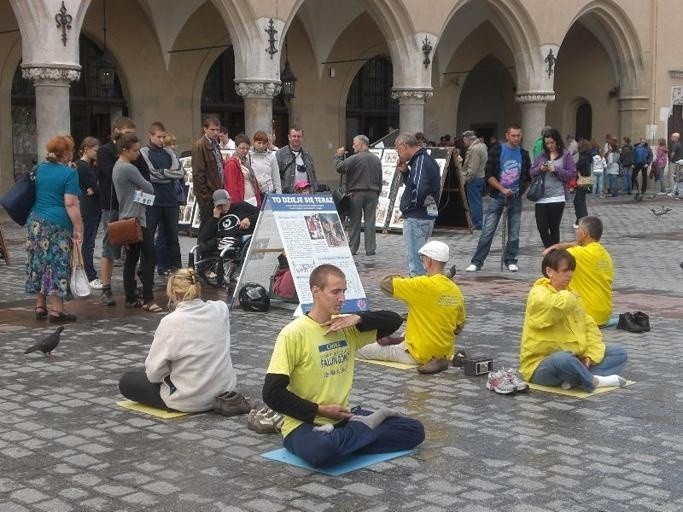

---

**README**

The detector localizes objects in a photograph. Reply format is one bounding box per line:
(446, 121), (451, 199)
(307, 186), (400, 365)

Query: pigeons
(661, 204), (672, 212)
(23, 325), (65, 358)
(650, 208), (667, 218)
(444, 264), (456, 279)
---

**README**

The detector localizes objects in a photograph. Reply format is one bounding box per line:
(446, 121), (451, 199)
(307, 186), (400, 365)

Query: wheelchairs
(187, 234), (252, 289)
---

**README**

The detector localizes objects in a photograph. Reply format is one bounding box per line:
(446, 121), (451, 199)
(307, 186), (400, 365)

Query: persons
(668, 132), (681, 200)
(191, 113), (319, 298)
(532, 125), (550, 163)
(138, 120), (186, 278)
(573, 139), (594, 228)
(119, 268), (236, 415)
(415, 130), (501, 204)
(529, 129), (577, 249)
(461, 130), (488, 231)
(541, 216), (615, 328)
(466, 122), (533, 272)
(260, 263), (425, 470)
(97, 115), (150, 307)
(393, 133), (441, 322)
(64, 136), (103, 299)
(354, 238), (465, 364)
(518, 247), (629, 391)
(111, 132), (163, 312)
(652, 138), (668, 195)
(565, 134), (653, 201)
(335, 134), (383, 256)
(24, 136), (85, 327)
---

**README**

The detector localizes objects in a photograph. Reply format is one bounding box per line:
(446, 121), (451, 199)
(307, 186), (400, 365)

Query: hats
(212, 189), (230, 206)
(418, 241), (449, 262)
(462, 130), (475, 138)
(294, 179), (311, 191)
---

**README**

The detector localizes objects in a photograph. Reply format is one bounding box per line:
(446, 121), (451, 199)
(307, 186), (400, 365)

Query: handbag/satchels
(0, 170), (35, 226)
(331, 186), (348, 215)
(69, 240), (91, 298)
(107, 218), (143, 246)
(526, 172), (544, 201)
(576, 176), (594, 186)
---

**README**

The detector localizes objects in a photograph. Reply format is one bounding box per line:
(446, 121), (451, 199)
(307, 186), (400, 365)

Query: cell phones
(543, 162), (547, 165)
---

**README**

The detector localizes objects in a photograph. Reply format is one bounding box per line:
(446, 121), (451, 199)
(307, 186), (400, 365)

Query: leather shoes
(632, 312), (649, 331)
(617, 312), (642, 332)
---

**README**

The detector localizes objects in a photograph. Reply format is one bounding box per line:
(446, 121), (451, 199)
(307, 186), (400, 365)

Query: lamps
(91, 0), (116, 91)
(280, 33), (297, 103)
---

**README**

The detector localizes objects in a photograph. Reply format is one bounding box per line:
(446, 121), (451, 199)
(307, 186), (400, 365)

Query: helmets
(238, 282), (269, 312)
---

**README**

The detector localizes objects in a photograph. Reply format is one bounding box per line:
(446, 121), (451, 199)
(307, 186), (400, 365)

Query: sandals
(34, 306), (47, 319)
(49, 310), (76, 322)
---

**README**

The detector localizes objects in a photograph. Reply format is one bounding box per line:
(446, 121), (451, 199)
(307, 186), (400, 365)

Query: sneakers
(416, 354), (448, 375)
(507, 264), (517, 271)
(485, 366), (530, 394)
(214, 390), (284, 434)
(465, 264), (478, 272)
(89, 267), (174, 313)
